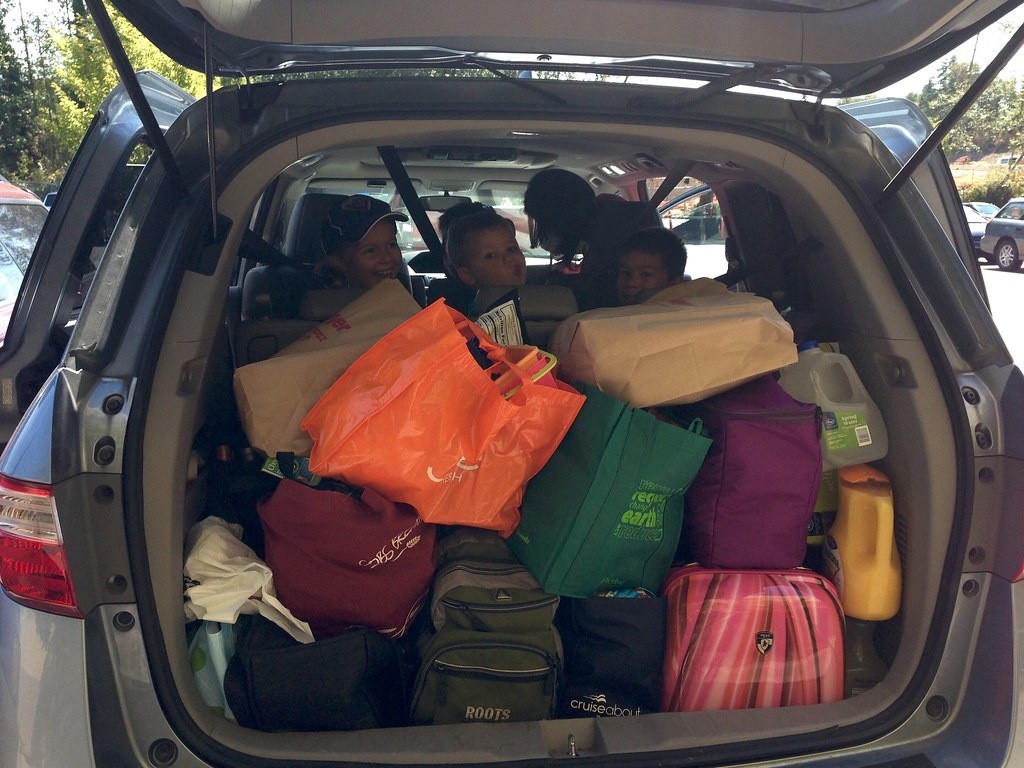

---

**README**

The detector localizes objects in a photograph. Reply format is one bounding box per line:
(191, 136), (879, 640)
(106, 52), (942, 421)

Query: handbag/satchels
(172, 267), (850, 716)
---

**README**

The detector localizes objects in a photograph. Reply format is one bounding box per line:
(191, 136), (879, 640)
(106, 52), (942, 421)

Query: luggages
(658, 560), (848, 712)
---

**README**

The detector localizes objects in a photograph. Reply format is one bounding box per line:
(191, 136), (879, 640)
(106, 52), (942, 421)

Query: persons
(318, 166), (687, 305)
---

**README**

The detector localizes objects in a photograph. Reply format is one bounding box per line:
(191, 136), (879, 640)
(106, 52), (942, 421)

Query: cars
(980, 195), (1024, 272)
(963, 202), (1012, 222)
(963, 205), (994, 264)
(43, 192), (57, 211)
(0, 174), (49, 345)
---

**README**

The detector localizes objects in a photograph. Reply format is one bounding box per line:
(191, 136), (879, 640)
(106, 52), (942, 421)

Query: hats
(321, 194), (410, 257)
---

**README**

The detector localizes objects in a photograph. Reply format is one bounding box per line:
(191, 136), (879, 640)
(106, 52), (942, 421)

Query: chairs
(236, 288), (364, 368)
(519, 284), (579, 346)
(240, 193), (349, 321)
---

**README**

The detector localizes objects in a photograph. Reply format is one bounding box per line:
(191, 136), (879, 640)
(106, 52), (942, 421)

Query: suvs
(0, 0), (1024, 768)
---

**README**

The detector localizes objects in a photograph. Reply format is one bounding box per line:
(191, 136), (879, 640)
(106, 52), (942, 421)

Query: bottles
(820, 465), (902, 621)
(776, 339), (889, 472)
